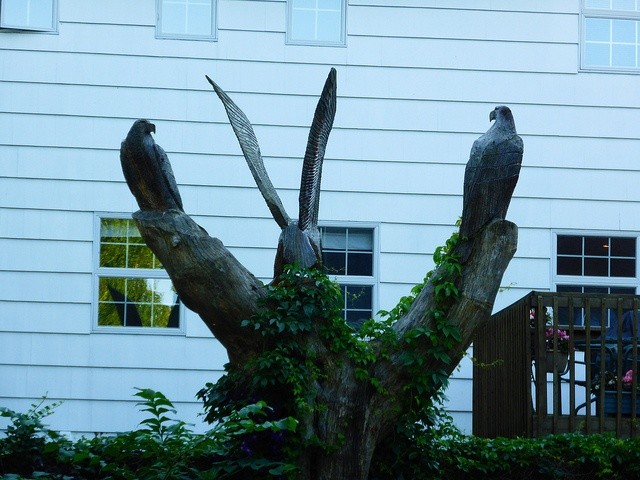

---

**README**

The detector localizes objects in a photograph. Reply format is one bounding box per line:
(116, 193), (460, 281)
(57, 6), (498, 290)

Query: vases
(599, 390), (632, 415)
(539, 350), (569, 373)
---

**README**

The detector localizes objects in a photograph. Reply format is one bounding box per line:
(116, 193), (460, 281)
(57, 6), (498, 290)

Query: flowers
(529, 303), (574, 350)
(592, 367), (639, 396)
